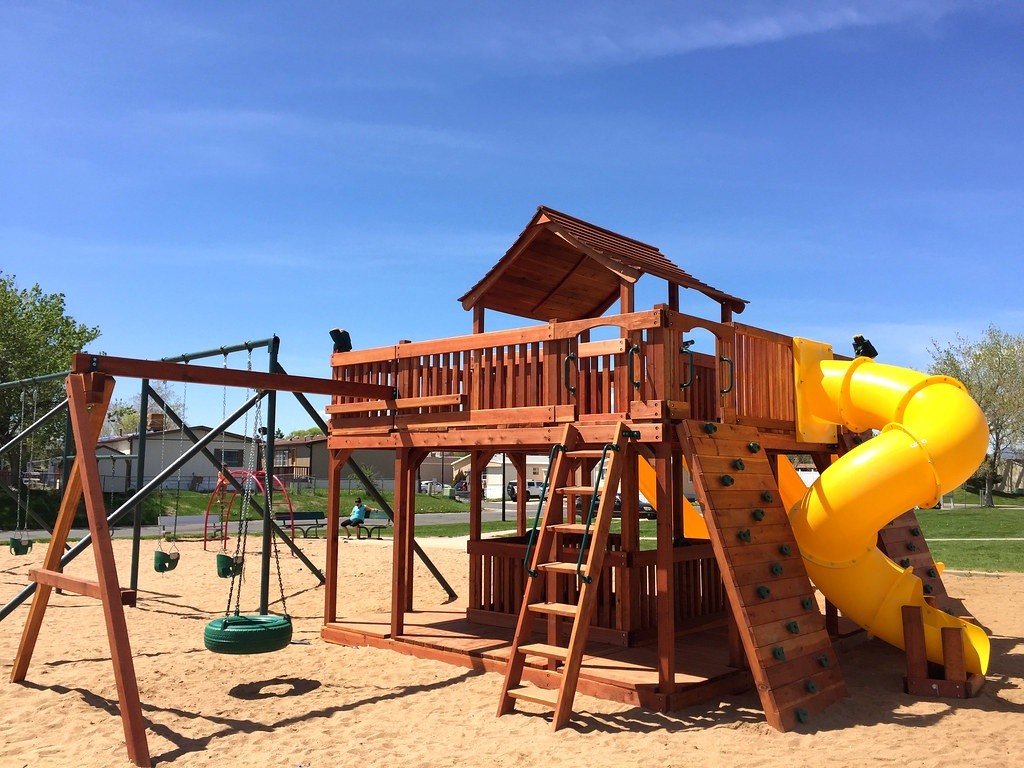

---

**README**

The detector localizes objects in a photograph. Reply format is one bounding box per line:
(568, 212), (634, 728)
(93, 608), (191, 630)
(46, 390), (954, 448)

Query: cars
(454, 481), (467, 492)
(539, 482), (549, 502)
(574, 492), (657, 521)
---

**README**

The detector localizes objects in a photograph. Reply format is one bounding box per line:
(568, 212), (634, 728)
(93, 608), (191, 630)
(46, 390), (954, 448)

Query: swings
(204, 392), (293, 655)
(154, 378), (187, 573)
(216, 353), (254, 578)
(9, 385), (38, 556)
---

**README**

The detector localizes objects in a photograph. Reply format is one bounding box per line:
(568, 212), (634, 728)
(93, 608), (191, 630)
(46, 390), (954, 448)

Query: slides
(788, 357), (993, 677)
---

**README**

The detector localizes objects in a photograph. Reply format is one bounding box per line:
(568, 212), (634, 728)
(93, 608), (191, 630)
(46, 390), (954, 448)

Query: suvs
(420, 480), (452, 495)
(507, 478), (547, 502)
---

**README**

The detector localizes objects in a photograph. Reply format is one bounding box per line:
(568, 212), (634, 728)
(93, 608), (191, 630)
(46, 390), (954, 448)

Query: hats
(355, 498), (361, 504)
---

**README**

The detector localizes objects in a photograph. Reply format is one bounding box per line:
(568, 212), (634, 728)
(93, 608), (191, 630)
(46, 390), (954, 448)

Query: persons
(340, 498), (374, 539)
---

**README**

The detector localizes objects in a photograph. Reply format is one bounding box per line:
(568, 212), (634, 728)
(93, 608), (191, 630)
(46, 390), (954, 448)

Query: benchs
(158, 516), (223, 538)
(275, 511), (327, 538)
(357, 510), (394, 539)
